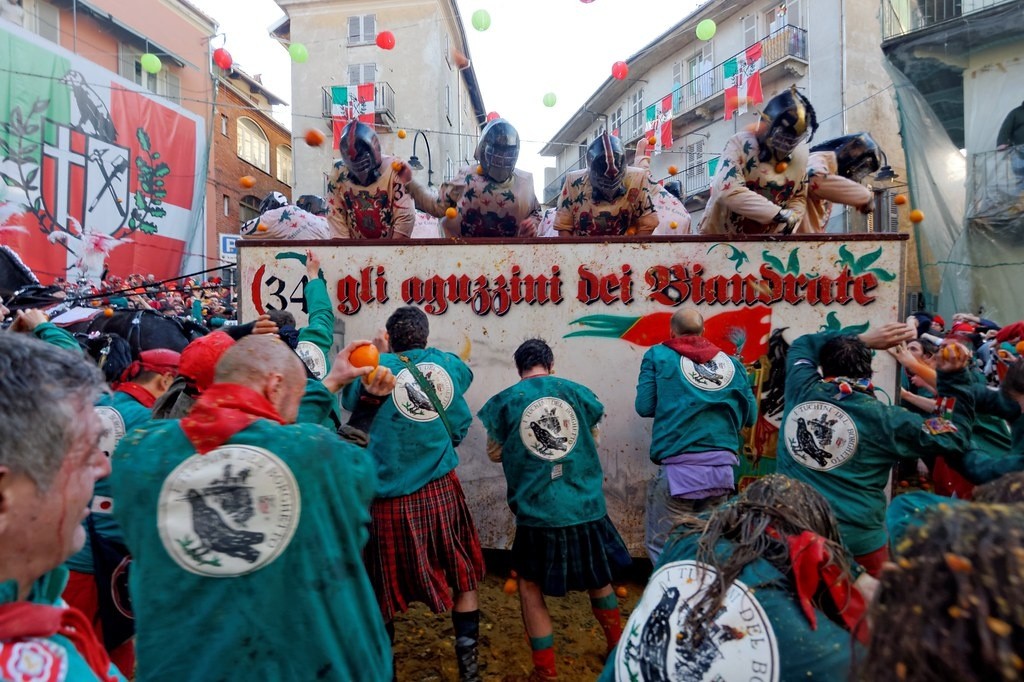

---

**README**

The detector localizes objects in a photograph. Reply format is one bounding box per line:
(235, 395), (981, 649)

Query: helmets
(836, 132), (882, 183)
(474, 118), (520, 182)
(758, 88), (819, 165)
(259, 191), (289, 215)
(339, 119), (382, 187)
(587, 130), (628, 202)
(297, 195), (322, 215)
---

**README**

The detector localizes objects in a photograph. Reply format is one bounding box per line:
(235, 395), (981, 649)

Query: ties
(1, 603), (119, 682)
(178, 384), (287, 455)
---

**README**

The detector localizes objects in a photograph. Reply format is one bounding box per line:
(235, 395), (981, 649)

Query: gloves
(772, 209), (799, 236)
(856, 197), (876, 215)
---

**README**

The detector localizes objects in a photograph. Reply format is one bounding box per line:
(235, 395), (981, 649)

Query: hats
(122, 348), (181, 378)
(933, 316), (945, 325)
(178, 331), (236, 392)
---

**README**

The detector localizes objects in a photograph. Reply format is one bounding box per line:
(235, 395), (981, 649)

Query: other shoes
(500, 669), (561, 682)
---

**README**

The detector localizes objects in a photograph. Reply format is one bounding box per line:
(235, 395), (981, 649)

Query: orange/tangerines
(91, 300), (114, 317)
(503, 569), (629, 598)
(238, 130), (1024, 388)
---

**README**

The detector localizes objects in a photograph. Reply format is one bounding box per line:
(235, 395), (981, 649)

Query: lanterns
(696, 18), (716, 41)
(214, 48), (232, 70)
(288, 43), (308, 63)
(141, 53), (161, 73)
(580, 0), (594, 3)
(487, 112), (500, 123)
(543, 92), (556, 107)
(376, 31), (395, 50)
(472, 9), (490, 31)
(612, 61), (629, 80)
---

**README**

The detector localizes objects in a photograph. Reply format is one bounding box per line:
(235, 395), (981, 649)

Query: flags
(644, 93), (673, 157)
(722, 41), (764, 122)
(331, 82), (376, 150)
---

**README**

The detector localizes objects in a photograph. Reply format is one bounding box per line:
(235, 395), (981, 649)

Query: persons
(696, 83), (882, 235)
(341, 306), (486, 682)
(0, 248), (395, 682)
(240, 190), (330, 239)
(326, 88), (692, 239)
(475, 338), (633, 682)
(597, 473), (1024, 682)
(635, 307), (1024, 630)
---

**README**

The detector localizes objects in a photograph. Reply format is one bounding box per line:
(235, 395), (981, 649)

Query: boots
(454, 642), (481, 682)
